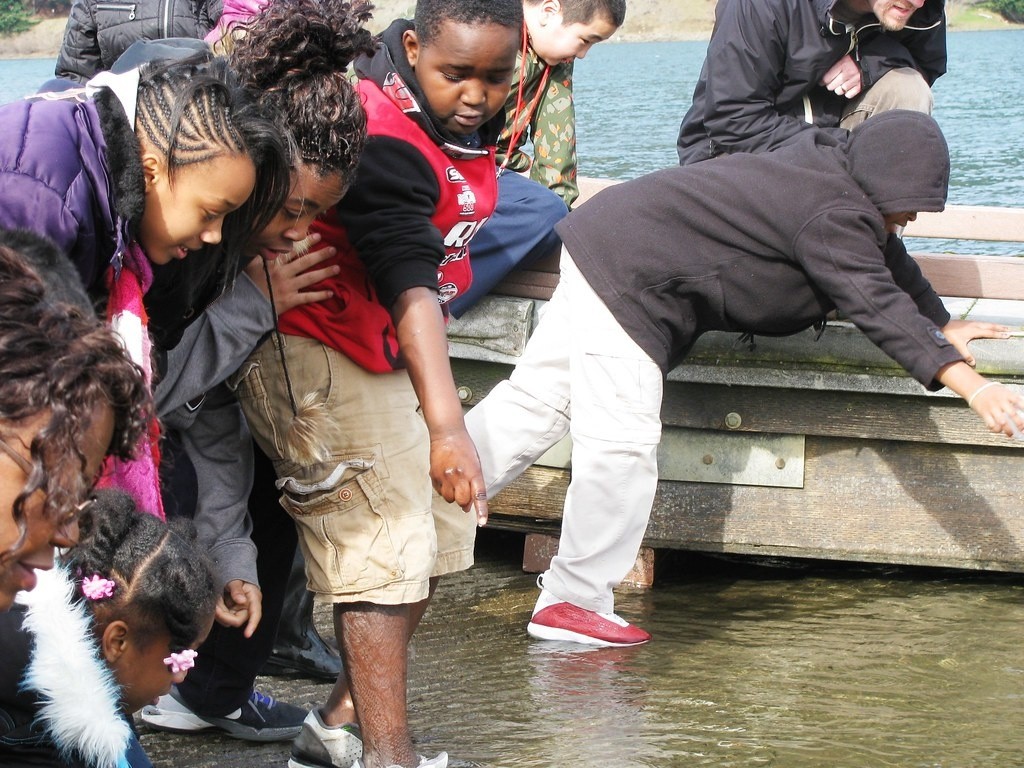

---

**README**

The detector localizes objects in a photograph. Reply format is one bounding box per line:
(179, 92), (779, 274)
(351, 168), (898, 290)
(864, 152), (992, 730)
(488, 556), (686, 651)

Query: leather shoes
(271, 625), (341, 677)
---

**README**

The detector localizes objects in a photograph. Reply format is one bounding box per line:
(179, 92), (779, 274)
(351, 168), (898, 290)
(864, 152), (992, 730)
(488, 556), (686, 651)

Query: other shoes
(526, 589), (651, 646)
(141, 681), (308, 740)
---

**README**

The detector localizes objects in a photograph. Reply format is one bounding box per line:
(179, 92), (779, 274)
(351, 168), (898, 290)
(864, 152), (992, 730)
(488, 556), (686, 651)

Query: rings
(841, 87), (846, 93)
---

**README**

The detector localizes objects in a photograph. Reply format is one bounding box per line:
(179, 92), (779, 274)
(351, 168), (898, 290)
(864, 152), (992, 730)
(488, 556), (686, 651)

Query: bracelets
(968, 381), (1002, 406)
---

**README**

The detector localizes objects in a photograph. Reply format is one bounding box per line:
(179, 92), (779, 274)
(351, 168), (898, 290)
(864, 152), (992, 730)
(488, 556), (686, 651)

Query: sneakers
(287, 707), (363, 768)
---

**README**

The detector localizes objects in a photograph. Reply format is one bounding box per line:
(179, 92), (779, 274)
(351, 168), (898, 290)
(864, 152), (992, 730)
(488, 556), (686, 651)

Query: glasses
(0, 441), (100, 524)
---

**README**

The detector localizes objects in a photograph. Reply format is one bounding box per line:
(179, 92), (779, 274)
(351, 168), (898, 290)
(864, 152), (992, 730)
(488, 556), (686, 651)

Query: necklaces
(496, 20), (549, 178)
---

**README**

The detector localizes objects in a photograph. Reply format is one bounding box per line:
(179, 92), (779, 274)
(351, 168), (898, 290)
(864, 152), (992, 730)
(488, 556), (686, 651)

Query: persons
(463, 113), (1023, 646)
(678, 0), (947, 163)
(0, 0), (379, 744)
(223, 0), (525, 768)
(494, 1), (626, 213)
(0, 226), (222, 767)
